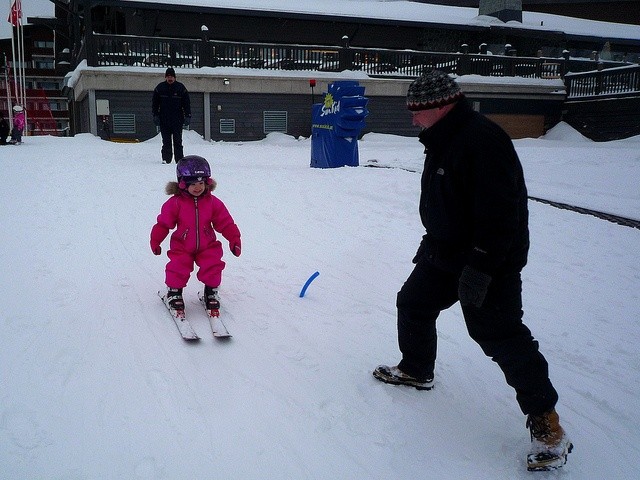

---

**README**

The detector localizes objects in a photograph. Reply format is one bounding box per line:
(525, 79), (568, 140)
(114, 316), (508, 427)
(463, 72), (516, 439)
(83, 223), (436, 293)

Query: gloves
(221, 223), (241, 257)
(456, 255), (500, 323)
(150, 222), (169, 255)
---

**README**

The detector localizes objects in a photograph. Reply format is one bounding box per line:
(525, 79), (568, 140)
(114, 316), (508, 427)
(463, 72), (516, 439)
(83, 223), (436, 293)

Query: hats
(164, 67), (177, 79)
(405, 67), (464, 112)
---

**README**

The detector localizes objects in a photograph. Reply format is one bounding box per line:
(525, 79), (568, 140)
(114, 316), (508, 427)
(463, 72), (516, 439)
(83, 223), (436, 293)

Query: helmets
(176, 155), (212, 197)
(12, 105), (26, 112)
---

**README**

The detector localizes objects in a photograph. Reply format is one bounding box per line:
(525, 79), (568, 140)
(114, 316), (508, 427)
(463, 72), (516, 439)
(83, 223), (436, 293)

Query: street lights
(309, 79), (317, 104)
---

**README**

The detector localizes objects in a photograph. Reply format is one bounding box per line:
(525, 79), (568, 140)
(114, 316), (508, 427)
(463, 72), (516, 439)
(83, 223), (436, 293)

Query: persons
(150, 155), (241, 311)
(372, 70), (574, 472)
(151, 67), (192, 165)
(10, 105), (25, 146)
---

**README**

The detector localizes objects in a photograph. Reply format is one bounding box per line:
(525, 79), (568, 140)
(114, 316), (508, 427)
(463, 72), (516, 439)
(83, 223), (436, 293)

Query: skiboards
(157, 289), (233, 341)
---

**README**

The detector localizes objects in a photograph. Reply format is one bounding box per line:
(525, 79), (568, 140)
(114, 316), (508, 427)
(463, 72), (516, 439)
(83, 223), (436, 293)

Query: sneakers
(166, 287), (185, 311)
(204, 285), (221, 310)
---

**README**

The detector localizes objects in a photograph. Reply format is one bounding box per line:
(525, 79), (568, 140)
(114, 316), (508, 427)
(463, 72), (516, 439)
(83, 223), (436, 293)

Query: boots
(525, 407), (575, 472)
(372, 364), (436, 391)
